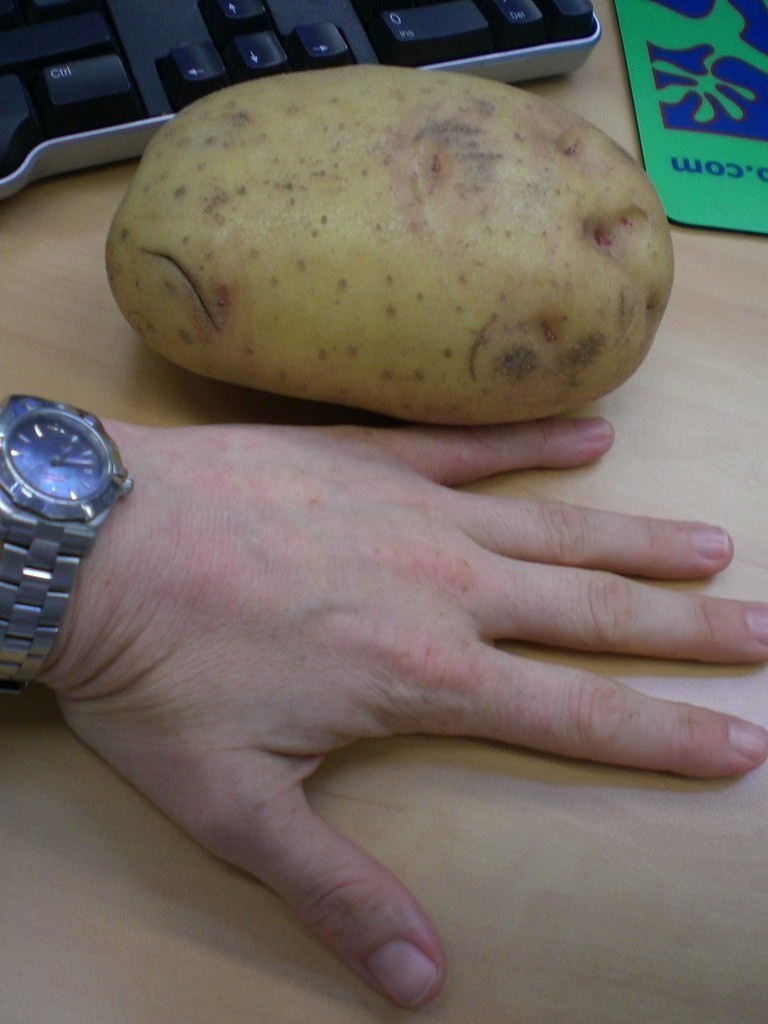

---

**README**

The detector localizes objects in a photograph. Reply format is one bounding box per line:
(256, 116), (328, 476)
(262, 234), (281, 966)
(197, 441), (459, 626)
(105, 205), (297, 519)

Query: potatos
(103, 61), (677, 426)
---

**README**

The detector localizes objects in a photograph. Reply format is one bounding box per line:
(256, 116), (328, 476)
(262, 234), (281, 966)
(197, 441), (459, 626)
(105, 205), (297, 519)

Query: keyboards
(0, 0), (601, 201)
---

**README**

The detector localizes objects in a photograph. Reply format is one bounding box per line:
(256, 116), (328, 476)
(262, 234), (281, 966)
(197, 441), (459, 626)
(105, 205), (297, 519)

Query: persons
(1, 393), (767, 1010)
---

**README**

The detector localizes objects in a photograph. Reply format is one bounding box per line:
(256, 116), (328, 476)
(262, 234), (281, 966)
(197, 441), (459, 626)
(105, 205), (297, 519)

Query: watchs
(0, 393), (135, 697)
